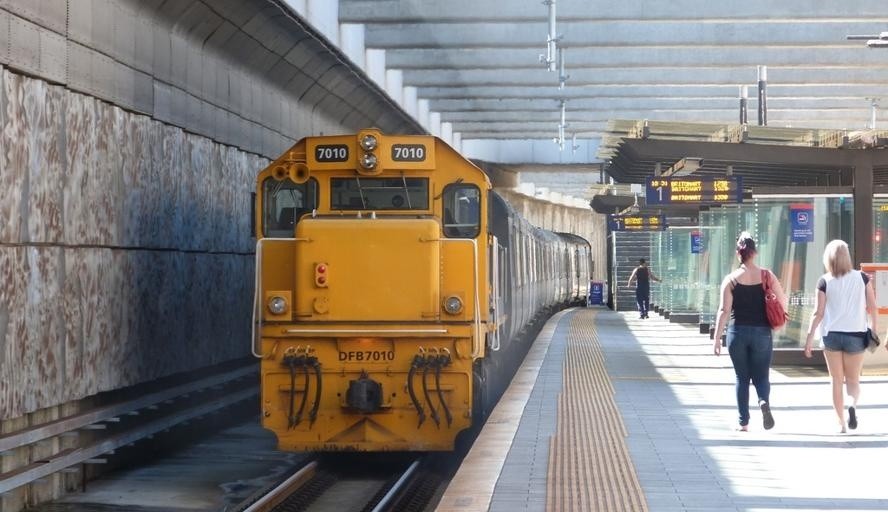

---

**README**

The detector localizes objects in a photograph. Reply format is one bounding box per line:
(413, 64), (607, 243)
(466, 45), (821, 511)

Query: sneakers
(735, 428), (747, 432)
(847, 405), (857, 430)
(760, 403), (774, 430)
(837, 426), (846, 433)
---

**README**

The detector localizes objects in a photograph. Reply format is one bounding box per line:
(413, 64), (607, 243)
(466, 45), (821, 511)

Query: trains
(245, 125), (593, 478)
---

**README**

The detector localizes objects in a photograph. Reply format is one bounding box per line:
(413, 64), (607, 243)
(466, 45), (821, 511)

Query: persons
(714, 233), (787, 433)
(626, 258), (662, 319)
(804, 240), (880, 434)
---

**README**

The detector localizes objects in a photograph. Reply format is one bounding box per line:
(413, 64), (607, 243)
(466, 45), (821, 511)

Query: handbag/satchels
(864, 328), (880, 353)
(765, 289), (786, 329)
